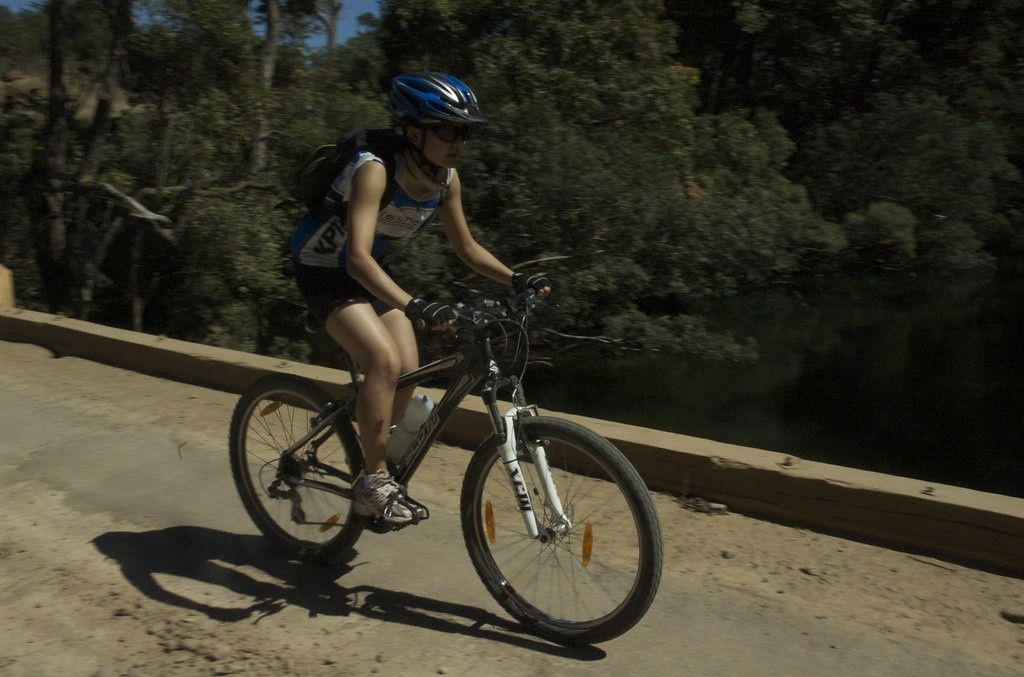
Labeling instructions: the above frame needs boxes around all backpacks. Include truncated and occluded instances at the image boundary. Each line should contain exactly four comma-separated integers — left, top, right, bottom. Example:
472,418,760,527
287,127,447,242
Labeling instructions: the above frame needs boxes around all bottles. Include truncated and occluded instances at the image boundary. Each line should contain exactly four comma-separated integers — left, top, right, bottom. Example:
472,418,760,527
385,394,434,466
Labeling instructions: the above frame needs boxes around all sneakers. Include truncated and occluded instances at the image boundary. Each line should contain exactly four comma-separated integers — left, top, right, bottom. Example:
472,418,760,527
352,469,423,523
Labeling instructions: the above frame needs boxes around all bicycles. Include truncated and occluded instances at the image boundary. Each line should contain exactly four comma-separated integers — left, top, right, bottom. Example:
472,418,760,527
227,287,664,651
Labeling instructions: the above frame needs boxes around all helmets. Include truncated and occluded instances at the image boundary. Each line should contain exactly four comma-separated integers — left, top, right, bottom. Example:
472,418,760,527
388,72,490,125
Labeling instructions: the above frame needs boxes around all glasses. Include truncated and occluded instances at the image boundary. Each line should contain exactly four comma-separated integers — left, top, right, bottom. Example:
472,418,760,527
431,124,472,142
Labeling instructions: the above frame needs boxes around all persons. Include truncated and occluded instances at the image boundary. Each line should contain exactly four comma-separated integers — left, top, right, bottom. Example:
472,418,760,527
292,70,552,529
